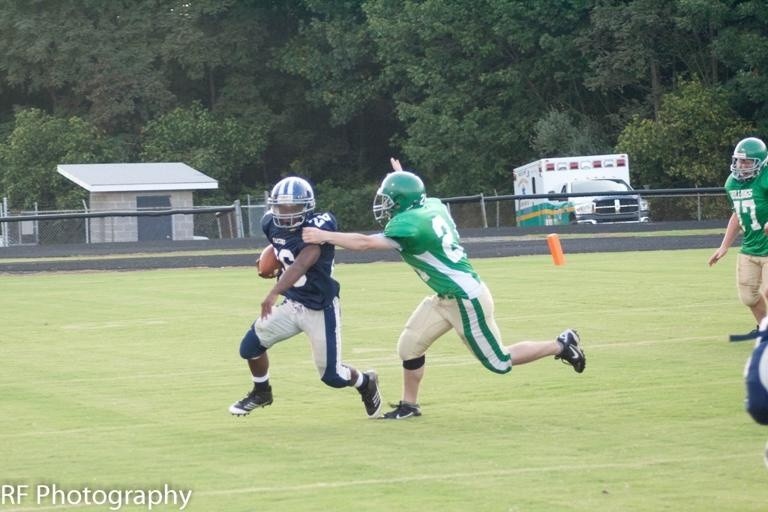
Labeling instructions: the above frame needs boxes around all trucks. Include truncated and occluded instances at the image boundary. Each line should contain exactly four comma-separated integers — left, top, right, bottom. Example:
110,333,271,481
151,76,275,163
513,153,650,225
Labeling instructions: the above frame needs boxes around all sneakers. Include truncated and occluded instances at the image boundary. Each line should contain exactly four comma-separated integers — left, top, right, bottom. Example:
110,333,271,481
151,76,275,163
554,328,586,375
381,400,422,420
357,369,383,419
228,385,273,417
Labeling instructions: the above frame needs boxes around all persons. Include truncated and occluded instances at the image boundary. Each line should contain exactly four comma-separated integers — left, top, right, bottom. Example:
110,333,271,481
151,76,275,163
302,157,585,419
229,176,383,418
730,317,768,467
709,137,768,326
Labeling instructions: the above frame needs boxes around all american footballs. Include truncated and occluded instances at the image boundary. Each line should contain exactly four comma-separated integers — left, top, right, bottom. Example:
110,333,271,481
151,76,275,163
259,244,283,275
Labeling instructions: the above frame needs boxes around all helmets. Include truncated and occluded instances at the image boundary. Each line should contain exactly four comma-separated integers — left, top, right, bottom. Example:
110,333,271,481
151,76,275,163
734,137,768,173
271,176,315,216
379,171,427,219
